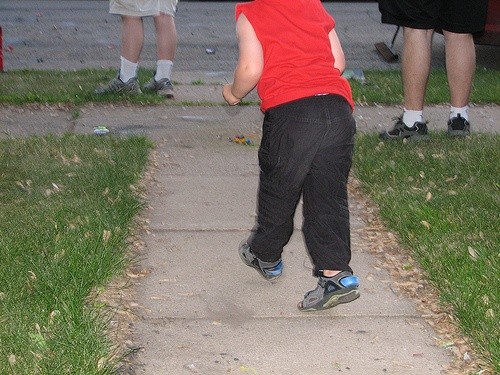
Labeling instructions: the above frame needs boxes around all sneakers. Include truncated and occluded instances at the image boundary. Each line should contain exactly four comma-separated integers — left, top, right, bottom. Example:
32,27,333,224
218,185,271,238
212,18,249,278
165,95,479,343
378,116,430,144
238,237,286,282
95,71,143,97
448,115,471,137
141,75,175,97
296,271,364,313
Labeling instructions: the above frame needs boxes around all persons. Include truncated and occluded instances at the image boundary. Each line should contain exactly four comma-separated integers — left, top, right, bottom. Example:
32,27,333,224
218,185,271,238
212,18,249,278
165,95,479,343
93,0,178,99
377,0,489,142
221,0,361,312
